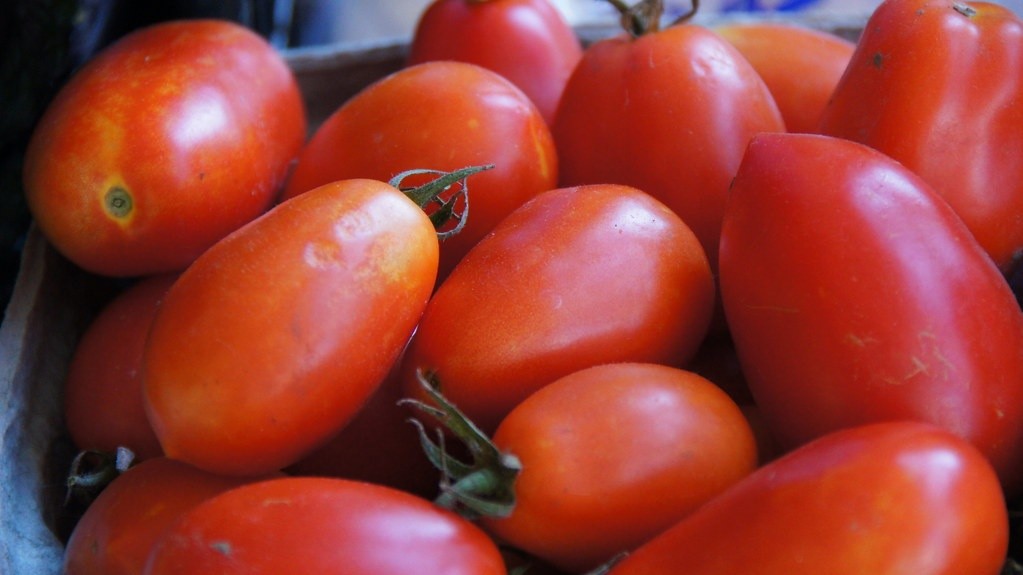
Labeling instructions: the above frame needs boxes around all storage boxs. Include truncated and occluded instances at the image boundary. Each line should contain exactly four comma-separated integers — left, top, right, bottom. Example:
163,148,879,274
0,8,1022,575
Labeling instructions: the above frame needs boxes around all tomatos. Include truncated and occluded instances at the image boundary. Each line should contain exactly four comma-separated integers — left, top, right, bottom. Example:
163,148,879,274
20,0,1023,575
